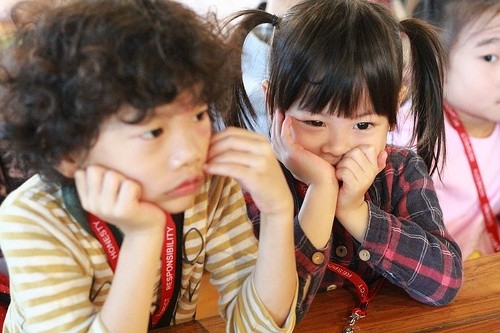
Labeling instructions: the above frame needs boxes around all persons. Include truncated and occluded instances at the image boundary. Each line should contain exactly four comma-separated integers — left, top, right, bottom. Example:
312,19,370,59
213,0,463,325
0,0,500,333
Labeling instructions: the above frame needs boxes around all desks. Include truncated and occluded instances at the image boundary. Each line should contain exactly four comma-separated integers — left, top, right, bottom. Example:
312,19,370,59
148,252,500,333
